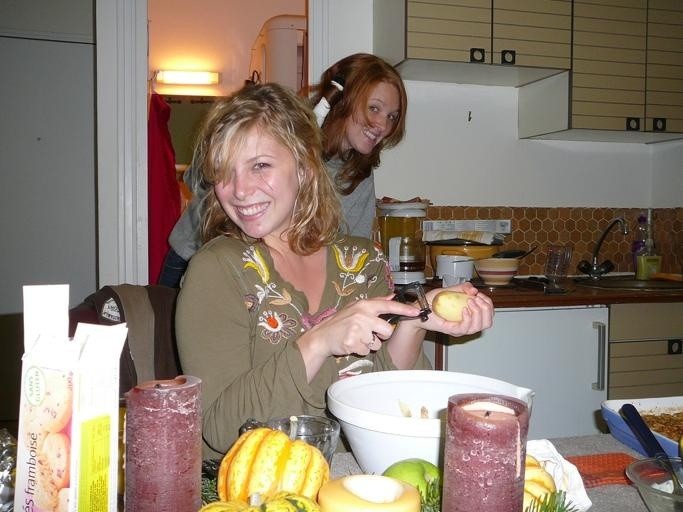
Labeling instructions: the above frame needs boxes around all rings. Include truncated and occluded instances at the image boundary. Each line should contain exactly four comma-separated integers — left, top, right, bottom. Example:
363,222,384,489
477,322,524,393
367,335,376,347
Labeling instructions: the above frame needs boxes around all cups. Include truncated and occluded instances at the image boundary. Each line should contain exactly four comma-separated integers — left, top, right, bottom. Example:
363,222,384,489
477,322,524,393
246,415,341,471
546,246,573,279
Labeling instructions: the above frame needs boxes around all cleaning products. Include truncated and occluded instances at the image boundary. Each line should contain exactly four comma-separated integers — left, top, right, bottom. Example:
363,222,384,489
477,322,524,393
634,225,663,280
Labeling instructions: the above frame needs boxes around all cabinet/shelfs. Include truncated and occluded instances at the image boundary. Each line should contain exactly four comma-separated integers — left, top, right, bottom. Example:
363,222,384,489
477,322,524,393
606,303,683,400
520,2,682,145
373,0,571,87
388,305,610,437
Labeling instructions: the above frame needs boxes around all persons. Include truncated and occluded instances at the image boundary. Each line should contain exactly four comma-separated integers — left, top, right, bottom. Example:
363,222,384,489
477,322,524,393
157,52,406,289
175,82,494,474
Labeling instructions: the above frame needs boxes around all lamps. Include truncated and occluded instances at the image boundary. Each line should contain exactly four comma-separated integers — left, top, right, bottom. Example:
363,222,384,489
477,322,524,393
153,69,221,85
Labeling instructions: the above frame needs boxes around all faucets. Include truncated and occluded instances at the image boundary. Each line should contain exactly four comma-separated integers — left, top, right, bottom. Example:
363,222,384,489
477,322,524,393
579,216,630,280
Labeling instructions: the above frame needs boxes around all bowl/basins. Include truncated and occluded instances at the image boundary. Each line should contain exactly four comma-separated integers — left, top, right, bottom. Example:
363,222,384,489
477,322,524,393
436,254,472,282
624,457,683,512
326,370,532,474
475,258,520,287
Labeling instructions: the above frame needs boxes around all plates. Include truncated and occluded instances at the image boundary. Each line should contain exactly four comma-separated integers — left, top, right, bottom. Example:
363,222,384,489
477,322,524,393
600,395,683,464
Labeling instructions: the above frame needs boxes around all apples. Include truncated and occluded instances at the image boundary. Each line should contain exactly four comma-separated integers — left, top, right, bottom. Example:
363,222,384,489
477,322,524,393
381,458,441,503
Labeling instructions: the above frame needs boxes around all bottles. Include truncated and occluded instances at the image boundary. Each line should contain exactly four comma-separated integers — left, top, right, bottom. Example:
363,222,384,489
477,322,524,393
628,214,649,277
632,238,662,274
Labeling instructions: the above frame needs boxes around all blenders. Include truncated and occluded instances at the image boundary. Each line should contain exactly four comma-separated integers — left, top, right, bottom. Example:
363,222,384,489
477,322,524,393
377,201,426,286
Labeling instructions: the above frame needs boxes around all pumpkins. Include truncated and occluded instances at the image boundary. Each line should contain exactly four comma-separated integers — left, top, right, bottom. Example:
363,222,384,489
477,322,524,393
522,455,556,512
217,428,329,505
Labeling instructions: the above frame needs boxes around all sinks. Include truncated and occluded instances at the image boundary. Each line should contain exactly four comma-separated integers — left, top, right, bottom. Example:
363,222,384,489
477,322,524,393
581,278,683,290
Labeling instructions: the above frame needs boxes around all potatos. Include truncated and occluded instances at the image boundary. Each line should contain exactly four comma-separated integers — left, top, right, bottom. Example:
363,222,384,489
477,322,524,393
430,290,478,324
